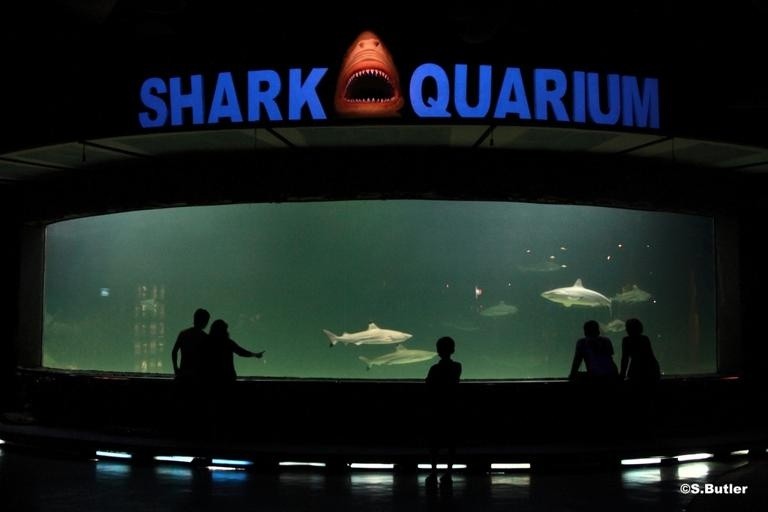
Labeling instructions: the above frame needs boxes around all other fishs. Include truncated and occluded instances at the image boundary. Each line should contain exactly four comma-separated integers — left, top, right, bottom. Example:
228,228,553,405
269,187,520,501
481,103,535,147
517,240,655,277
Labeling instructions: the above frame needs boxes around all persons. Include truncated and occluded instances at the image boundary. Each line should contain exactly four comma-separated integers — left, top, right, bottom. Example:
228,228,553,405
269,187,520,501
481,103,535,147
171,307,208,391
424,335,462,485
567,318,620,384
620,318,662,386
197,318,267,386
